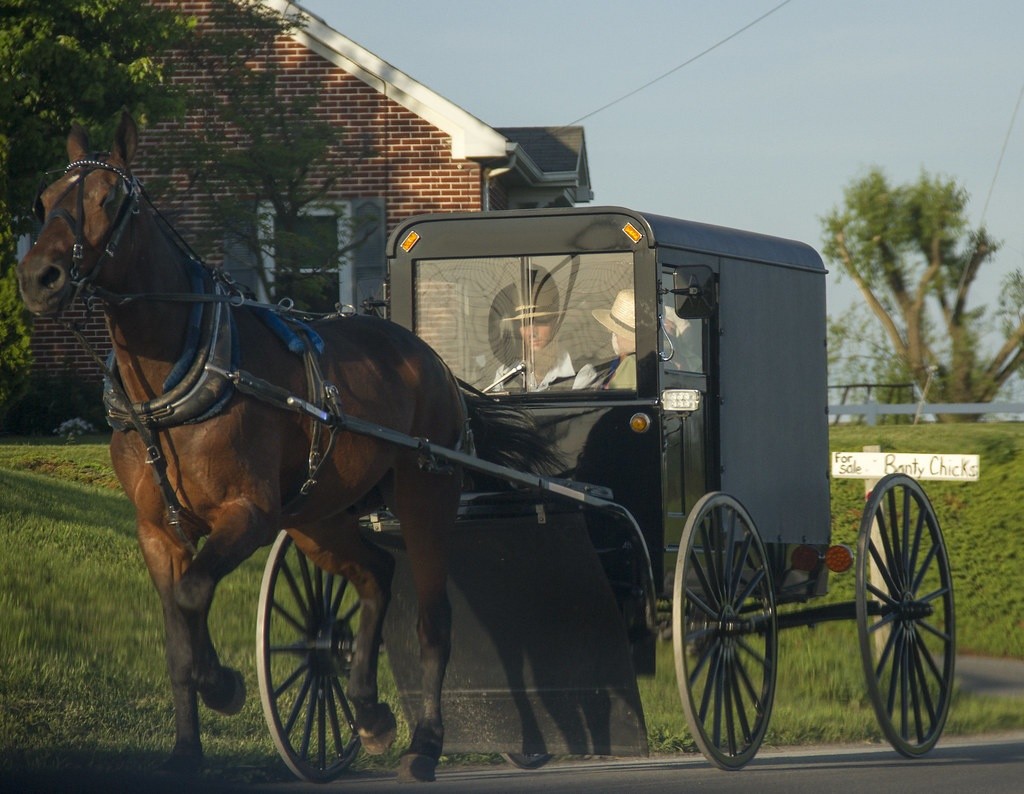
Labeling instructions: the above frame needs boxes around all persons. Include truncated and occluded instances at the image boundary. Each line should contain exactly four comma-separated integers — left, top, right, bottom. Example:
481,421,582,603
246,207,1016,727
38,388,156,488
662,306,703,373
572,288,637,391
473,288,587,392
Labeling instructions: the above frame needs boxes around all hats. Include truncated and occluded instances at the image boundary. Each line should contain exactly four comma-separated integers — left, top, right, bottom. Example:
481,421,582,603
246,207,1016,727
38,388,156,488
591,289,636,339
494,285,566,321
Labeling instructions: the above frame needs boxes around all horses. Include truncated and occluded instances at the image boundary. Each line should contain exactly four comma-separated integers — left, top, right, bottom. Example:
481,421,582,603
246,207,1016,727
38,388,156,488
14,107,572,794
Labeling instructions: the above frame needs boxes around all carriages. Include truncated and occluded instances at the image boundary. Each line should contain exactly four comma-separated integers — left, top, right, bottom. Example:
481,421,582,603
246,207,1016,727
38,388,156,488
22,108,956,782
